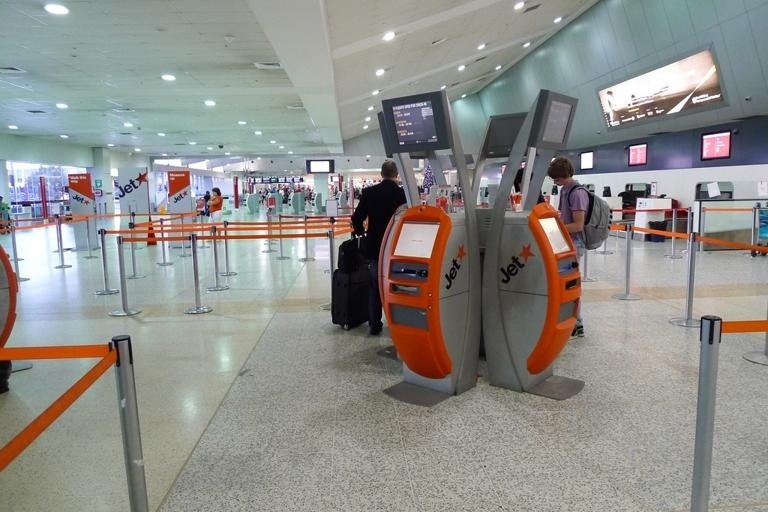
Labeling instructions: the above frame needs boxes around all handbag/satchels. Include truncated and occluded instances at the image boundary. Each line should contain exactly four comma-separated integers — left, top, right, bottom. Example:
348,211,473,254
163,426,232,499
337,230,370,273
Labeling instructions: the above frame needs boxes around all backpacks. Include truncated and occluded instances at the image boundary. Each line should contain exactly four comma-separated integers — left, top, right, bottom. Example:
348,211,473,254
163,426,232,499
565,184,614,250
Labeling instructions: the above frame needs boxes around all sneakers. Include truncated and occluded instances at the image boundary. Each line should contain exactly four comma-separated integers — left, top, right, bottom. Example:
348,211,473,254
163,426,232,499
569,325,586,339
370,321,384,335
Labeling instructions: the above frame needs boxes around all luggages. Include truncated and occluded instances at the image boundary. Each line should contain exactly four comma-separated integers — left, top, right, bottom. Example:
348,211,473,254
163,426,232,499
331,267,370,331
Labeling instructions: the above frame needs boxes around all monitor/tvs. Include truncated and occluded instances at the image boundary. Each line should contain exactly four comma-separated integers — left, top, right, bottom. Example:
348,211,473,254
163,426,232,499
484,112,529,159
501,157,558,175
528,88,578,150
579,151,593,170
628,144,646,166
306,158,336,175
377,111,429,160
700,130,732,161
380,90,453,156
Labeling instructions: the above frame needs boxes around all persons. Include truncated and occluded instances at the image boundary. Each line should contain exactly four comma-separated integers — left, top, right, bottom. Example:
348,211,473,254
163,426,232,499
351,161,408,336
514,168,545,206
547,157,590,340
0,196,12,234
207,187,224,242
256,179,373,205
416,182,490,199
203,191,211,216
